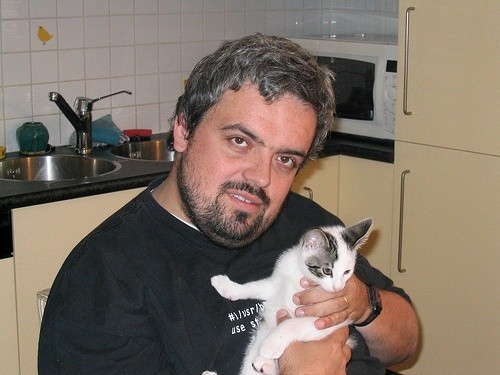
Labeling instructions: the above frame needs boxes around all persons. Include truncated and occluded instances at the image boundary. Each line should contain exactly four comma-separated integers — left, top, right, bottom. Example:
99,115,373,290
37,34,421,375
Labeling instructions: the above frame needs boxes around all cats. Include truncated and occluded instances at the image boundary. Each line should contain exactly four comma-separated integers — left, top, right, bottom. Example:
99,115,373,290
198,218,373,375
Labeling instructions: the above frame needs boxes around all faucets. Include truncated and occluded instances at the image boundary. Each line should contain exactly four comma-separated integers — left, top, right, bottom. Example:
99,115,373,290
47,90,95,156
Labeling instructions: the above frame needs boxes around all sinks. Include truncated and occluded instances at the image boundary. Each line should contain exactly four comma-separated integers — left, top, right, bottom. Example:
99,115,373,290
0,153,122,185
107,137,182,163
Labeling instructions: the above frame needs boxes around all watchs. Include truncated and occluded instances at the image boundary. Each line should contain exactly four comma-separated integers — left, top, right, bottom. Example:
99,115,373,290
355,284,382,327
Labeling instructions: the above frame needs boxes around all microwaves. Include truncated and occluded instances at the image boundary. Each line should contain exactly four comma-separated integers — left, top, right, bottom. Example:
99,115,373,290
282,32,399,141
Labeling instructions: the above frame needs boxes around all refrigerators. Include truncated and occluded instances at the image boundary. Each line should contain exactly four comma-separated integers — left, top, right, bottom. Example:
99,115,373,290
375,0,500,375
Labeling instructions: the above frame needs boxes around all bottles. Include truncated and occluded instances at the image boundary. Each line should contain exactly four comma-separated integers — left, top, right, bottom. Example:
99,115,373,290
16,122,49,152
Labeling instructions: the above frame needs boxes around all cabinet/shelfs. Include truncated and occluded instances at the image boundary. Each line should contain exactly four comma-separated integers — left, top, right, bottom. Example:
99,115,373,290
0,0,500,375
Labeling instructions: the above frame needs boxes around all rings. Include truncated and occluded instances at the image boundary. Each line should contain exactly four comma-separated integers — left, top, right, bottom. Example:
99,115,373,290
344,297,347,308
